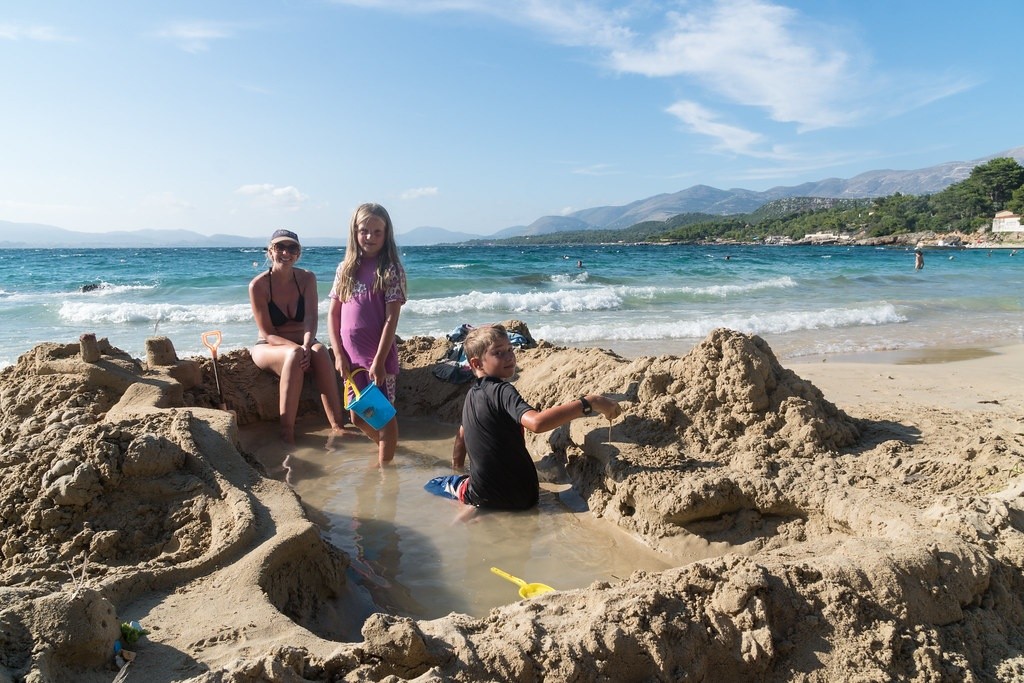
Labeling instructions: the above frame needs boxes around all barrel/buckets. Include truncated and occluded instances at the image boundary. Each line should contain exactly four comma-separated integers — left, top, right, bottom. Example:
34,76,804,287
344,368,398,431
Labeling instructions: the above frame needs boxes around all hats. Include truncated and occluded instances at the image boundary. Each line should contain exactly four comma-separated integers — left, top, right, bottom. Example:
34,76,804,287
271,229,298,246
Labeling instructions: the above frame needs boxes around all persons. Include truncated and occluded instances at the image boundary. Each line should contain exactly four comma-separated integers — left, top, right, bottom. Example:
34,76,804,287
915,250,924,269
574,261,584,267
328,203,408,470
247,229,343,432
421,323,622,525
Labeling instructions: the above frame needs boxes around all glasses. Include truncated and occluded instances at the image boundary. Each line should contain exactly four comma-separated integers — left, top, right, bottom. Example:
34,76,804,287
273,244,299,255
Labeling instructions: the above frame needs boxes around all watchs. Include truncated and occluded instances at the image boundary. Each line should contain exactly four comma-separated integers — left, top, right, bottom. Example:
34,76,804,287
579,397,592,415
